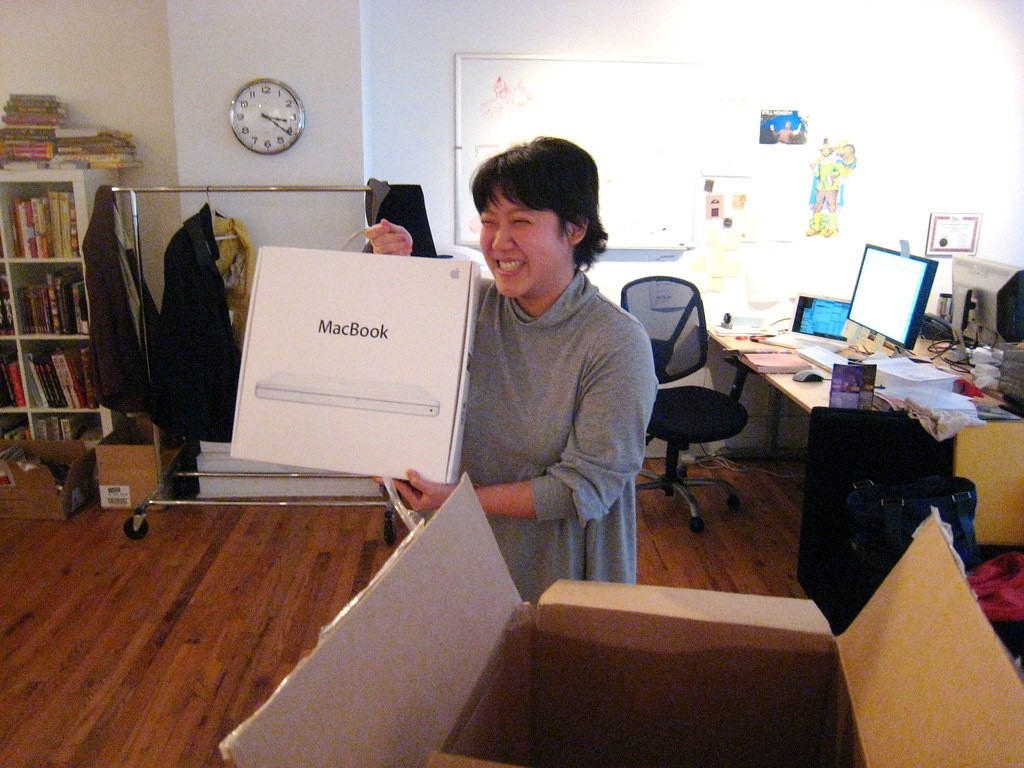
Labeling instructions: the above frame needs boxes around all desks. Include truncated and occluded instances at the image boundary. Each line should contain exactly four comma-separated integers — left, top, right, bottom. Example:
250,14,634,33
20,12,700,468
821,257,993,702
706,326,1024,464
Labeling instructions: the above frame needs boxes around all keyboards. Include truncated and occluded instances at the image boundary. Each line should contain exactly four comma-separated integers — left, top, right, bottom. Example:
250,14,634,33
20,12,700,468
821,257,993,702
796,346,850,373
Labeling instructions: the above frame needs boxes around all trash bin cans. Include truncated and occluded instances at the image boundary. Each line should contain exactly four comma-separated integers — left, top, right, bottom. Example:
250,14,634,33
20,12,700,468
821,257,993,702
798,409,951,636
845,475,981,614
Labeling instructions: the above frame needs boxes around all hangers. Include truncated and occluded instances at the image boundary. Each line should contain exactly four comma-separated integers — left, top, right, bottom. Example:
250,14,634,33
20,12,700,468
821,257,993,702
207,185,238,242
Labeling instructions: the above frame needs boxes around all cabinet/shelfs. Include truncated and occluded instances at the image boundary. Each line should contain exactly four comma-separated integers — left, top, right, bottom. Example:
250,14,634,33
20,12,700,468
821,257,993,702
1,169,121,451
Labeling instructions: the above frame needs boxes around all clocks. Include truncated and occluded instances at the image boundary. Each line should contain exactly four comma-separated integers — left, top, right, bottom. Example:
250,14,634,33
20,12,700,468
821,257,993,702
228,76,305,155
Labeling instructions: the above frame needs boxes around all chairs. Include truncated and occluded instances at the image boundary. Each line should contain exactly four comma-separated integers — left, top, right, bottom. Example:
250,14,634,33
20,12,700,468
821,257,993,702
794,408,1024,658
619,275,748,535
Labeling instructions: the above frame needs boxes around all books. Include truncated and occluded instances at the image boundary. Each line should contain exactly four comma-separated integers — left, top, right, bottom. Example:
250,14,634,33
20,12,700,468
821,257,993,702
1,94,143,486
723,347,811,373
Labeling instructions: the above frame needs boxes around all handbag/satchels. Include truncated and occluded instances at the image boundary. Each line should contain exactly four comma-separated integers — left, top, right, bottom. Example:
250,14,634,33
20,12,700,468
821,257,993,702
232,226,481,484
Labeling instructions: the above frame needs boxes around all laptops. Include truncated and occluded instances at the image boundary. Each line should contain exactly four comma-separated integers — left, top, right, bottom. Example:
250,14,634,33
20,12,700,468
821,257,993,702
762,293,852,353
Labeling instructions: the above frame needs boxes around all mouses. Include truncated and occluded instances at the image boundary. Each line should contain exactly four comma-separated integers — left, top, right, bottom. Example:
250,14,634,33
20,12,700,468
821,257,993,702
793,369,824,382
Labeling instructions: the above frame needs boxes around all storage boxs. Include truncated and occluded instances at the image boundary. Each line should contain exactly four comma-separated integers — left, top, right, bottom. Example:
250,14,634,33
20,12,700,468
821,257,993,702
95,416,178,512
230,244,481,485
0,438,96,521
216,472,1023,768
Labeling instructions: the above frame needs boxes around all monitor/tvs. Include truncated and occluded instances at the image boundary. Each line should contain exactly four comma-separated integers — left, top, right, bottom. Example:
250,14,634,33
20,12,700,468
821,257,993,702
847,244,939,351
944,255,1024,365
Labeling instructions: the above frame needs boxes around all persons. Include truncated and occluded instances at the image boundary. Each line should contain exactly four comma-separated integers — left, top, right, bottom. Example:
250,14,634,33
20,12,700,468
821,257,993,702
364,137,659,606
